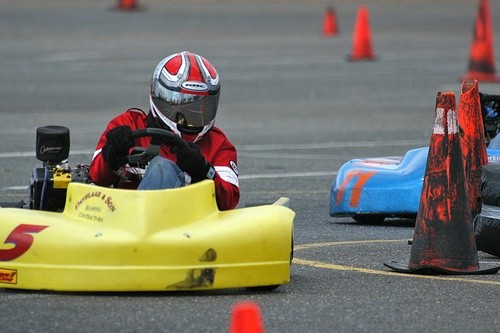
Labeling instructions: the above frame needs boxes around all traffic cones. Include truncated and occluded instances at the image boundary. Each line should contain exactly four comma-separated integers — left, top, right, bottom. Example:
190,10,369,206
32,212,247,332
460,0,499,82
345,6,377,61
230,302,265,332
322,6,339,37
112,0,139,10
385,78,499,275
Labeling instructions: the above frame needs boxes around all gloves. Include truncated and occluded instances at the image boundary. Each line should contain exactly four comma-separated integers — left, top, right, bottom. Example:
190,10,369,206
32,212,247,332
171,142,215,184
102,125,135,171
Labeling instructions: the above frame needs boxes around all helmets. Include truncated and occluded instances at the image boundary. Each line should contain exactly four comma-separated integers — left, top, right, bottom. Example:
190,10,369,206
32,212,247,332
149,51,220,143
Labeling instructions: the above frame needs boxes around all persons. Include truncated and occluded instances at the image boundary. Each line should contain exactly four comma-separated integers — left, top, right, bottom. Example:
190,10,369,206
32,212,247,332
89,50,240,211
